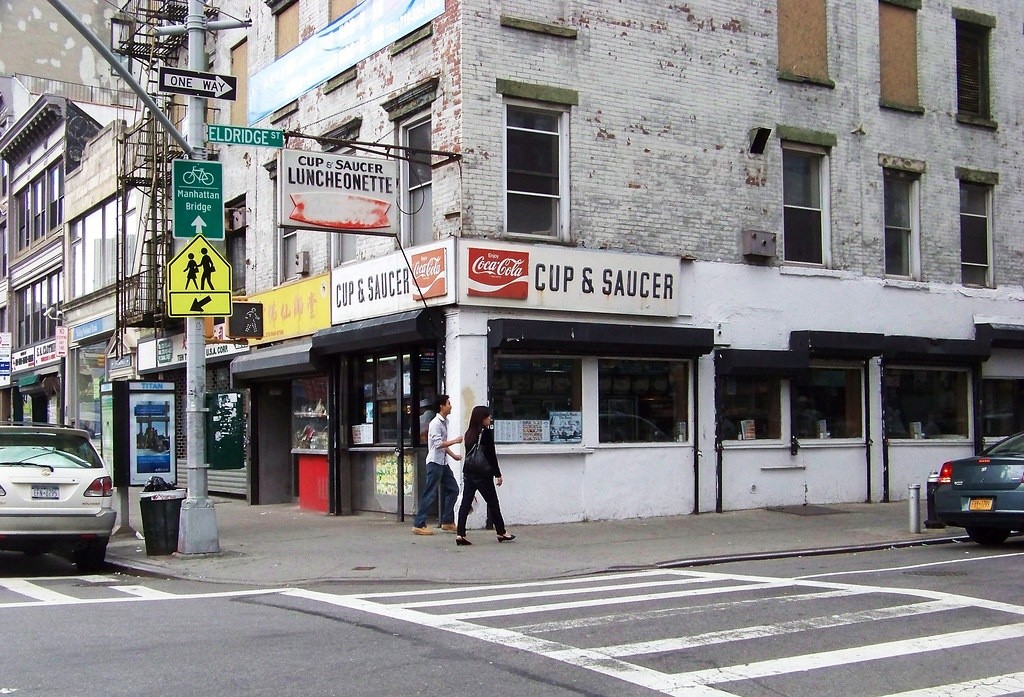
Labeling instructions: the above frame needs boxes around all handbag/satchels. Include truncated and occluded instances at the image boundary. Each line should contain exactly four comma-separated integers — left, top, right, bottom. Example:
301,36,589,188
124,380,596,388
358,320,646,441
463,429,492,476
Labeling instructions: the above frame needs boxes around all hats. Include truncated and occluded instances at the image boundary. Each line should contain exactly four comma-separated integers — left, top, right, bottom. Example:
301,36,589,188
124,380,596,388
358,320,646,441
420,398,432,407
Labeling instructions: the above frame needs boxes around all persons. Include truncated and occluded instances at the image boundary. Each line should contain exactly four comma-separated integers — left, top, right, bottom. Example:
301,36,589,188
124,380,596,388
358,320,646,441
456,405,517,546
411,395,464,534
408,399,437,446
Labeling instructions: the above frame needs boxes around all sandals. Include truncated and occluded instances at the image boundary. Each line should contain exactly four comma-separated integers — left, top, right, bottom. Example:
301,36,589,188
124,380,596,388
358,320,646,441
496,532,516,543
455,535,471,546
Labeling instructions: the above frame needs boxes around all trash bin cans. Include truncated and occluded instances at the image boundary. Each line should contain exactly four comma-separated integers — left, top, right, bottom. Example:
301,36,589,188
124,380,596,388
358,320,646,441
138,489,187,557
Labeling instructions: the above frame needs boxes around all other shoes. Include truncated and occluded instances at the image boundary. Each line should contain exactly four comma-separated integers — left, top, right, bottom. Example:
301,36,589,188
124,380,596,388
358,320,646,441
441,522,458,531
412,526,434,535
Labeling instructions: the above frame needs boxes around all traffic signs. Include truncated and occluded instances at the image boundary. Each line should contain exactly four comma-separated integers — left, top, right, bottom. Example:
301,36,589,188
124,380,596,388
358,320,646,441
157,65,239,102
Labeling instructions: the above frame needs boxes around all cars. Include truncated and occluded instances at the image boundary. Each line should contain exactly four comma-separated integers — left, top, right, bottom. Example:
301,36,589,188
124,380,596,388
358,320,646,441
0,421,118,575
932,431,1024,546
